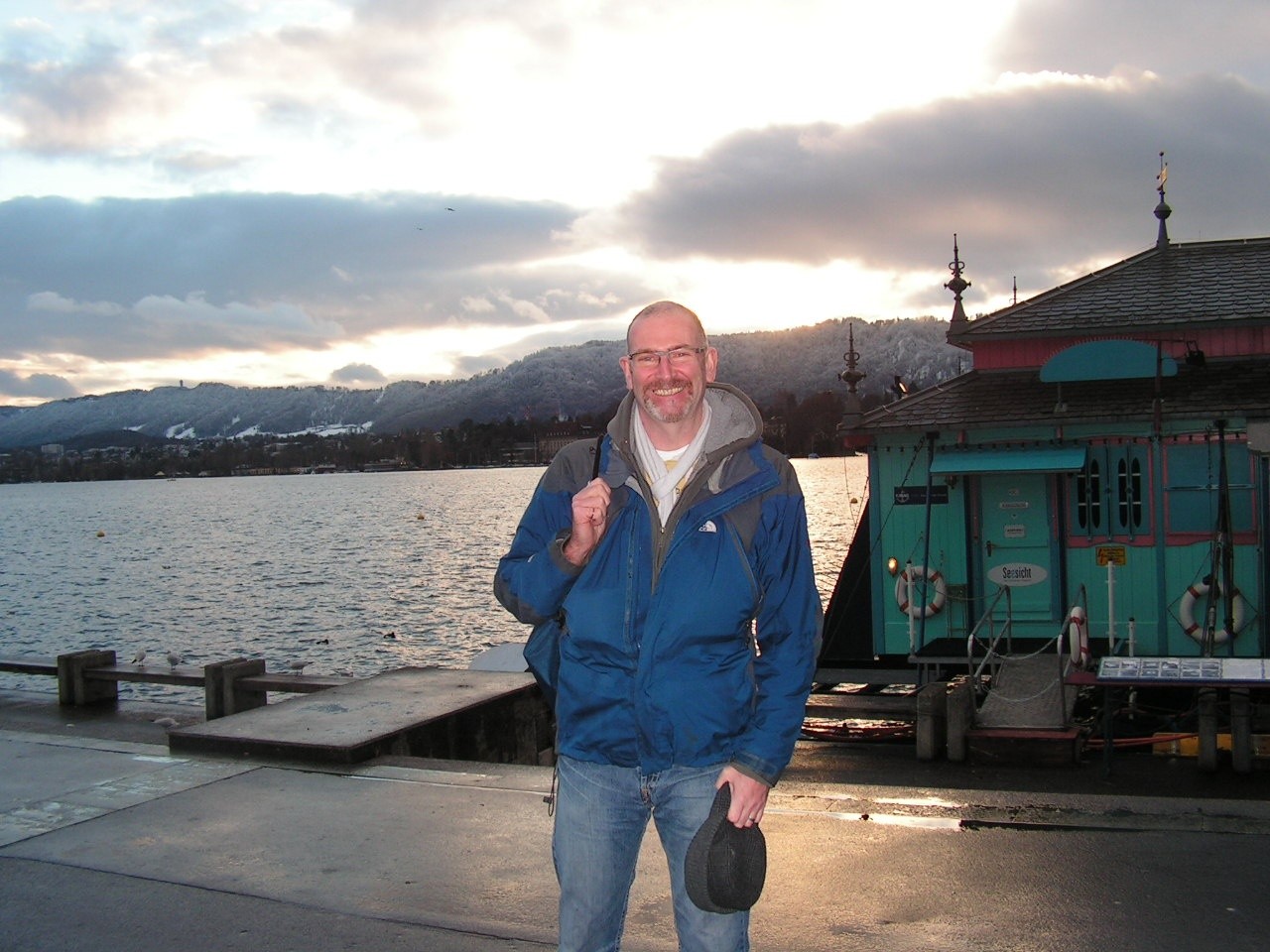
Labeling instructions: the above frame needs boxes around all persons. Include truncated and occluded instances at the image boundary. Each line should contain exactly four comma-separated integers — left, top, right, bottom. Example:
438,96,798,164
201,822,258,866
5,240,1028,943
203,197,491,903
493,300,824,951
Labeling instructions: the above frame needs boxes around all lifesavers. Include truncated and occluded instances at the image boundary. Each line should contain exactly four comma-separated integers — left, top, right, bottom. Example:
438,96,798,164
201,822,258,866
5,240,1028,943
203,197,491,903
895,566,946,618
1069,607,1088,668
1180,582,1244,643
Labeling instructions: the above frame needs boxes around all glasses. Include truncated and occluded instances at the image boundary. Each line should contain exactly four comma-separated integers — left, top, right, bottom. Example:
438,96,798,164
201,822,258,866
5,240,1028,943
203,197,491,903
628,346,708,370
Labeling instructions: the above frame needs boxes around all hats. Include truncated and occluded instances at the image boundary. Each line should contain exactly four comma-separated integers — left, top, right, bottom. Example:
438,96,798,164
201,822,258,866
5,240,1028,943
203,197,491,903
684,781,768,914
